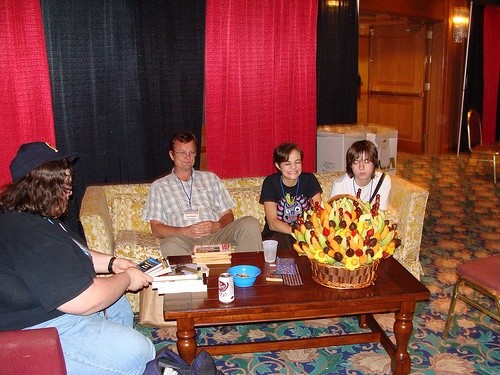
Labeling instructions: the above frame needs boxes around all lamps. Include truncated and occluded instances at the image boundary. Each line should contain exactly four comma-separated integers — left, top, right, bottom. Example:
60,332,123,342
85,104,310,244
453,7,470,43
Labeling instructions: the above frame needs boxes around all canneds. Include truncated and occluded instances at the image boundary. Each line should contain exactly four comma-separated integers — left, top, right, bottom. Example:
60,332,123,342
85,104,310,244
217,273,235,304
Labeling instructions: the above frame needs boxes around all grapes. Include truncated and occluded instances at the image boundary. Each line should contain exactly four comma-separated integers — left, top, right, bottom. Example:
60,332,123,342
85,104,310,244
295,220,401,261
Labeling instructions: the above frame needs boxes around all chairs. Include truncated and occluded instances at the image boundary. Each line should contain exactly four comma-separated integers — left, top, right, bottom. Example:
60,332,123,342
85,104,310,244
465,108,500,184
0,327,67,375
442,254,500,339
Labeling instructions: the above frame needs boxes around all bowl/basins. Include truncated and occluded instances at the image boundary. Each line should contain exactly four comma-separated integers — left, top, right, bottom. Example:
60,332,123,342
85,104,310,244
226,265,261,287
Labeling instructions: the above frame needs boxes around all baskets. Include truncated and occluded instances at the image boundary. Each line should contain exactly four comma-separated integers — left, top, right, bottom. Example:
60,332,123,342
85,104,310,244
309,194,381,290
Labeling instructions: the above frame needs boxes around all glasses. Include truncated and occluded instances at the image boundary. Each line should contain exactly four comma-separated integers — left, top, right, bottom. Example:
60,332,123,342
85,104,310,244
172,151,197,159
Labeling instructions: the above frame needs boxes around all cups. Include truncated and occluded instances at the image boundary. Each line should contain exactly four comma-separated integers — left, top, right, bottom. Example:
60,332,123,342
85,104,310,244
262,240,278,263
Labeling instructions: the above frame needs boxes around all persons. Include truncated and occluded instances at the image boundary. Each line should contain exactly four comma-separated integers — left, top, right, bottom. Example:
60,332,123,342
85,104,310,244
141,130,263,260
259,143,323,238
328,140,392,211
0,142,156,375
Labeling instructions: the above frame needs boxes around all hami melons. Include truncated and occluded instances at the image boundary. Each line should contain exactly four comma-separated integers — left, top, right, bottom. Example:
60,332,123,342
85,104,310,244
294,201,396,271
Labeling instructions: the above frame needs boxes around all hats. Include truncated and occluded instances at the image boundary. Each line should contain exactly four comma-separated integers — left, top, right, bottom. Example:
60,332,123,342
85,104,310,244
9,142,80,183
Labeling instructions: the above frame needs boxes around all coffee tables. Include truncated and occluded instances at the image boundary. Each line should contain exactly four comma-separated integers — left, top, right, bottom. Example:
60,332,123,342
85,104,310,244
164,249,431,375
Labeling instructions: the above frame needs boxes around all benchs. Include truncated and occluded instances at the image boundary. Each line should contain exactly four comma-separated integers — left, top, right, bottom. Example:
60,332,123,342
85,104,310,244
78,170,429,317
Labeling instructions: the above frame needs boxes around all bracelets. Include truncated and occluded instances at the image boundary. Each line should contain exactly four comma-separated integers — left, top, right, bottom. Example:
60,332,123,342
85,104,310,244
108,257,116,272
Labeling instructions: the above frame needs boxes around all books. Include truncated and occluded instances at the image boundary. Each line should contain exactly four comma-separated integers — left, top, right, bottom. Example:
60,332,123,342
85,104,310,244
151,264,209,294
191,243,232,264
135,256,172,277
